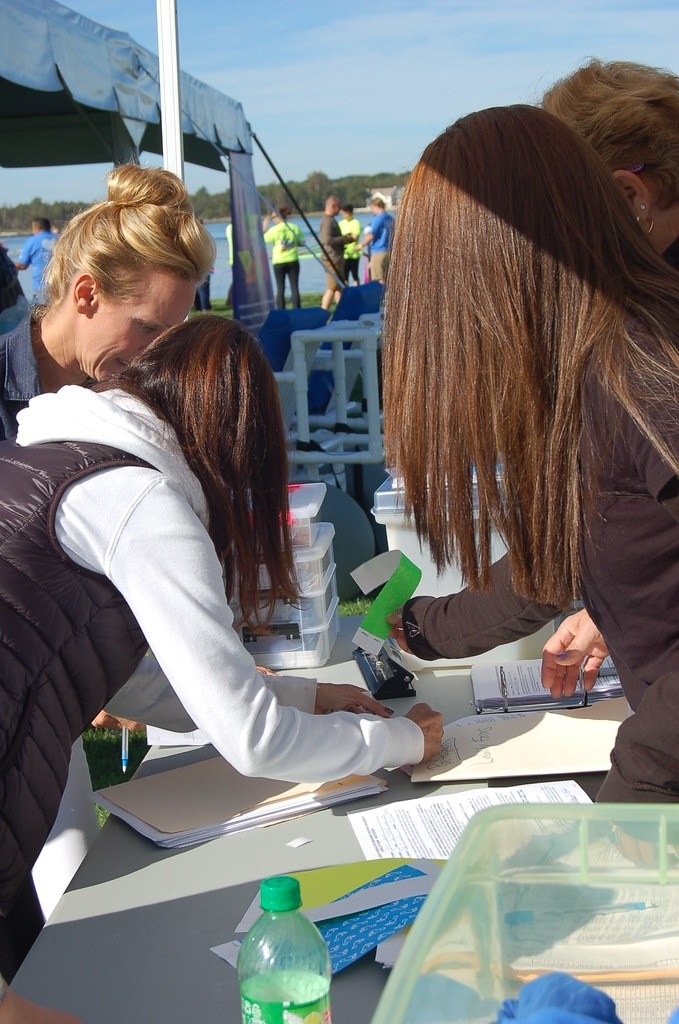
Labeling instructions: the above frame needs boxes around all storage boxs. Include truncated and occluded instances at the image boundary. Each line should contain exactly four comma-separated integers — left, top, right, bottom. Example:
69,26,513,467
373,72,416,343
369,471,556,669
364,802,678,1024
234,486,342,671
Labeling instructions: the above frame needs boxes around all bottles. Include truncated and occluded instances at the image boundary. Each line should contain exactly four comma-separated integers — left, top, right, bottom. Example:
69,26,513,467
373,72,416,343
235,876,332,1024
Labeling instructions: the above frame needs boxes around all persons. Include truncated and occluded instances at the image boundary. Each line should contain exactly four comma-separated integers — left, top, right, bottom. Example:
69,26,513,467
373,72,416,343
1,316,443,984
383,104,678,868
0,163,215,438
225,198,394,308
0,217,61,333
541,59,679,698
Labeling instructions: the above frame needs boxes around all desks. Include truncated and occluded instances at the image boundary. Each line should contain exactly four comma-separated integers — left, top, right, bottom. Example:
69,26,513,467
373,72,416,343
0,618,679,1024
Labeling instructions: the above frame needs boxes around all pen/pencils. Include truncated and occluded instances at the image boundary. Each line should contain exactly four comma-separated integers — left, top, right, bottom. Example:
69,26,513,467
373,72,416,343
392,626,418,634
499,666,509,711
503,900,657,924
120,726,129,774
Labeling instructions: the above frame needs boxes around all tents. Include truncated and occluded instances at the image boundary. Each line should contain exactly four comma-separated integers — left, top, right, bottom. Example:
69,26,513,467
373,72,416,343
0,0,348,288
